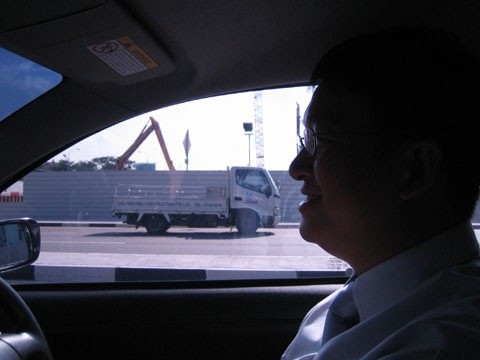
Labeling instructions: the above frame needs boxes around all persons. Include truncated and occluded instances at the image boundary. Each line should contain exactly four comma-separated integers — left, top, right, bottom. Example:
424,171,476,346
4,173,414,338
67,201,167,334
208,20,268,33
280,25,480,360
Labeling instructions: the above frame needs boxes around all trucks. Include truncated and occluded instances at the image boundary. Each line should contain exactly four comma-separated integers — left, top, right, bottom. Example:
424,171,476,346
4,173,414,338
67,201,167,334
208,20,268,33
113,167,283,236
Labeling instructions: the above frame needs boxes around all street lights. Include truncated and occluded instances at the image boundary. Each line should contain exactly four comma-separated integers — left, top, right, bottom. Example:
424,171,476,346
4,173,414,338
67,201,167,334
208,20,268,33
242,121,254,167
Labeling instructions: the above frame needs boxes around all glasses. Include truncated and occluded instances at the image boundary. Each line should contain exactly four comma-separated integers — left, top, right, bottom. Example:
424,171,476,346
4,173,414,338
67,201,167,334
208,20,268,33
296,128,381,156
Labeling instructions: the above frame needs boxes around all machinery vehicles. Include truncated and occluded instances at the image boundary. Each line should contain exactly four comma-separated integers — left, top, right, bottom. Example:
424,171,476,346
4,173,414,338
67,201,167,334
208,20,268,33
115,117,176,173
